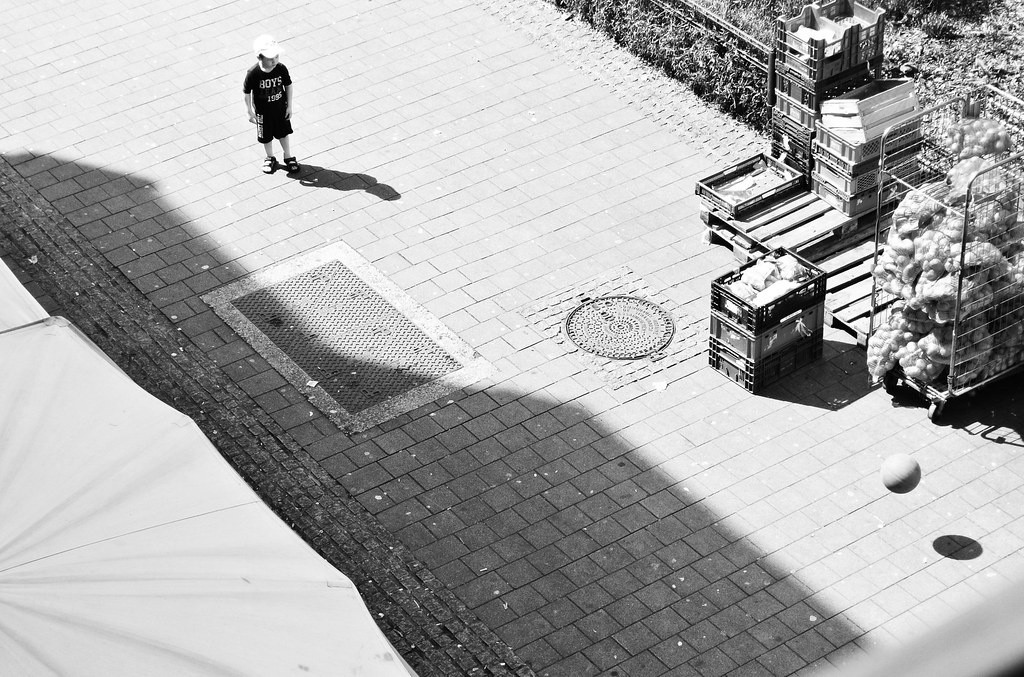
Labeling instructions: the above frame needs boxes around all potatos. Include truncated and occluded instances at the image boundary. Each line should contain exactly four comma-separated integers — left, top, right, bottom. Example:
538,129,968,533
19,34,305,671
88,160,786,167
867,121,1023,386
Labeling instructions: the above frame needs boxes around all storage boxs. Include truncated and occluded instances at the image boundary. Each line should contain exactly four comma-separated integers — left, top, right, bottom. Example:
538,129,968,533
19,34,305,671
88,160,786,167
711,245,827,337
710,302,824,363
708,328,824,394
694,0,922,220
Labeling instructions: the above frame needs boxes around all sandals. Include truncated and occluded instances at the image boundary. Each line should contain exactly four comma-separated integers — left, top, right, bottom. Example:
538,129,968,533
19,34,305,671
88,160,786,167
283,156,300,173
263,156,276,173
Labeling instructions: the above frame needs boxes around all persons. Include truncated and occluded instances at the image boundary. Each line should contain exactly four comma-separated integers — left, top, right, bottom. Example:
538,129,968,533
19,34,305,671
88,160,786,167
242,35,301,174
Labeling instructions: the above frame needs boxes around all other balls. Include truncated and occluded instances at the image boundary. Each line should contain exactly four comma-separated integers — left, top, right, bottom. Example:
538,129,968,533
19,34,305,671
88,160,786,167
880,453,922,495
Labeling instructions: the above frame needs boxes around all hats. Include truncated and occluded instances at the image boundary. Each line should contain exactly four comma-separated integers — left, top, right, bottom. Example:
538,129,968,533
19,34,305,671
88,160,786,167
254,36,281,58
